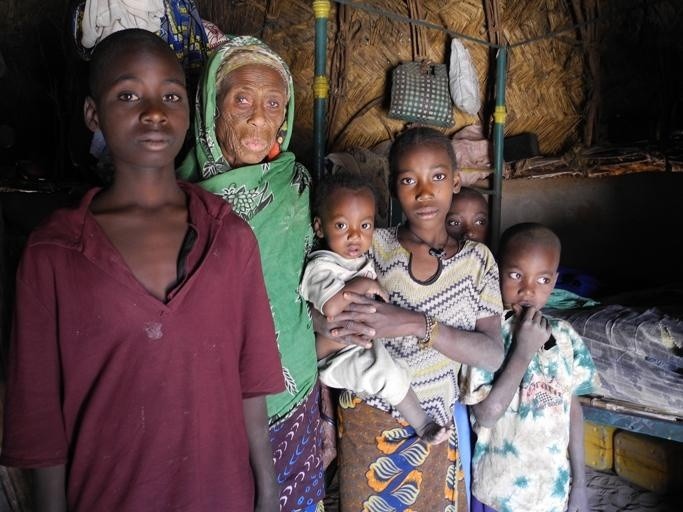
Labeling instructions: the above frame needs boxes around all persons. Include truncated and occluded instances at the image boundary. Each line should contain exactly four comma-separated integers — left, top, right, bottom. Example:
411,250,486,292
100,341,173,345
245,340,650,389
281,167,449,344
295,172,456,447
310,125,507,511
0,26,290,510
445,186,491,243
469,222,603,511
173,33,340,510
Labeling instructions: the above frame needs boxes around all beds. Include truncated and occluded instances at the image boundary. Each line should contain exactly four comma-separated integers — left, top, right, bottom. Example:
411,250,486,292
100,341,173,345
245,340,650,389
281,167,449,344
311,0,682,448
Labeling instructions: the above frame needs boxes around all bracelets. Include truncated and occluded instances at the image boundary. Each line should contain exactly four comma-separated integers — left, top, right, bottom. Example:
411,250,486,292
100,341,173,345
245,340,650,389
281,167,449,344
320,414,337,427
416,311,440,354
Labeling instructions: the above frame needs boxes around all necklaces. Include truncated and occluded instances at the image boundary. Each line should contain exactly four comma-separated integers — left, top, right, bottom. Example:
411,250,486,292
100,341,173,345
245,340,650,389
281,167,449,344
405,228,450,258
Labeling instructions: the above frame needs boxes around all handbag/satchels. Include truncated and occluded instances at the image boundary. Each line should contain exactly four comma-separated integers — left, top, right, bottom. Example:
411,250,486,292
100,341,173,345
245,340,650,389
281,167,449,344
446,40,483,114
389,60,457,130
